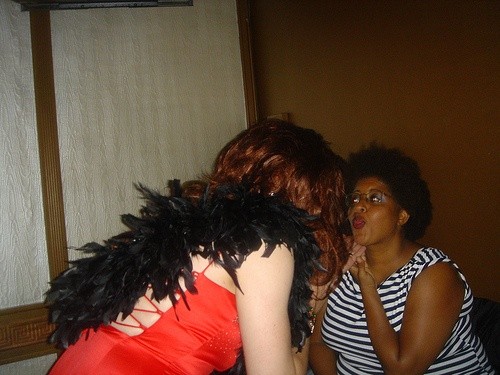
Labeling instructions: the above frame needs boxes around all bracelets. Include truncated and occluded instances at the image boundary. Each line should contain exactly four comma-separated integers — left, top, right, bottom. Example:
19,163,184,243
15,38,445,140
307,307,316,332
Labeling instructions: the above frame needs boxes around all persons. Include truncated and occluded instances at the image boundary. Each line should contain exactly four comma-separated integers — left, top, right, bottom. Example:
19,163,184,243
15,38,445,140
308,145,497,375
47,118,354,375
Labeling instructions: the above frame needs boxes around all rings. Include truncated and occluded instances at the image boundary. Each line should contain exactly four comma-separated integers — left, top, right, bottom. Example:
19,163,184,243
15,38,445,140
348,252,355,256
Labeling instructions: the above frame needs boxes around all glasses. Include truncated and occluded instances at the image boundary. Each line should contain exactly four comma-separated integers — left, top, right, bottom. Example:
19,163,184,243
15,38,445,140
344,190,393,208
